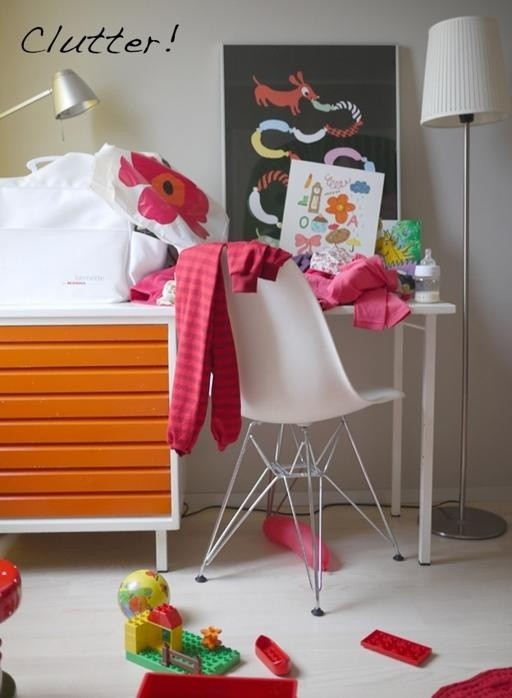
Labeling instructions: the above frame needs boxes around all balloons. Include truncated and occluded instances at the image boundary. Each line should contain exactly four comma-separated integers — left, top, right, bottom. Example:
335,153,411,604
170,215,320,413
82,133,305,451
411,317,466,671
262,515,330,571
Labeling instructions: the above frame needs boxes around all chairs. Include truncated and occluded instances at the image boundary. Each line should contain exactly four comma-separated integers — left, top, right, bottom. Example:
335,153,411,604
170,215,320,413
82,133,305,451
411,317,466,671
174,240,407,620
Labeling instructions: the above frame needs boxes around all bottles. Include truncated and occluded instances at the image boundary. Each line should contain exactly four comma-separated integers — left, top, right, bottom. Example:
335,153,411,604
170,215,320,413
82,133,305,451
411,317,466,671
413,248,441,305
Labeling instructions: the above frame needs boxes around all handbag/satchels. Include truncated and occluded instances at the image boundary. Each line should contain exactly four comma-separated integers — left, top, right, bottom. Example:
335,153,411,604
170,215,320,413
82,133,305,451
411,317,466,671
0,144,168,310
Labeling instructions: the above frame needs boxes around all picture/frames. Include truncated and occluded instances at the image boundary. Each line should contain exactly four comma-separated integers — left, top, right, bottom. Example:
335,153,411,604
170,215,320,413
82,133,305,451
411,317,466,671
218,41,400,244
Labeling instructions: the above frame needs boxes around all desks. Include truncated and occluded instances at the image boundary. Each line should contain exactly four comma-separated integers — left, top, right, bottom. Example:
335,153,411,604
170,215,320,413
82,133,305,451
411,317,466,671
1,299,456,568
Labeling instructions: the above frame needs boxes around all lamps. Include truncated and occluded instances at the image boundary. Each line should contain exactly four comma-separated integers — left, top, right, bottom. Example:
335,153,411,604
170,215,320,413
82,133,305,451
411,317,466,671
420,12,511,543
1,67,99,124
